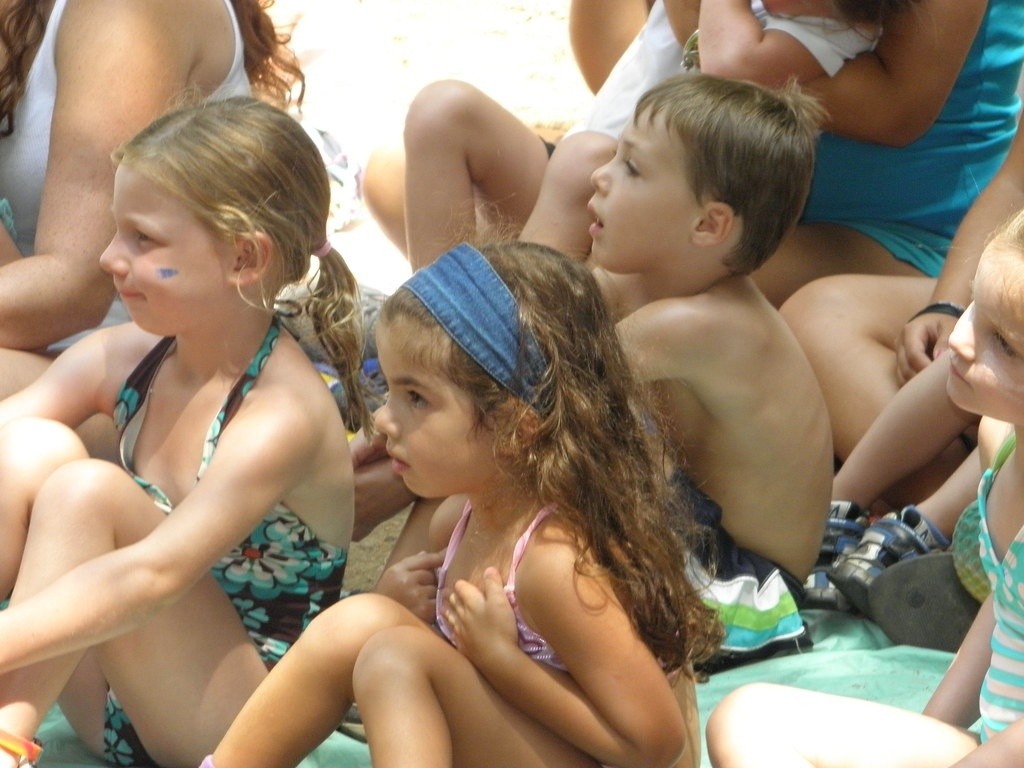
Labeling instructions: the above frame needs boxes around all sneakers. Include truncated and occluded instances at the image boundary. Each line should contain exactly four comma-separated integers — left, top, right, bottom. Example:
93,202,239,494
802,499,870,610
826,505,950,612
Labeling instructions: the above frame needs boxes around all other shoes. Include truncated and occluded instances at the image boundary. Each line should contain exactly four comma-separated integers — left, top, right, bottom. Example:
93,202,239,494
312,359,392,443
867,551,983,652
286,281,393,360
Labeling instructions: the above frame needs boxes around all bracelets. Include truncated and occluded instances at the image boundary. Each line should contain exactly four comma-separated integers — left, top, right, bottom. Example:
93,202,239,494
910,302,968,317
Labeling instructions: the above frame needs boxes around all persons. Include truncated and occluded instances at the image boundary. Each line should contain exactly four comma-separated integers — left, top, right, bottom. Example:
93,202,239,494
195,239,728,768
0,0,1024,768
